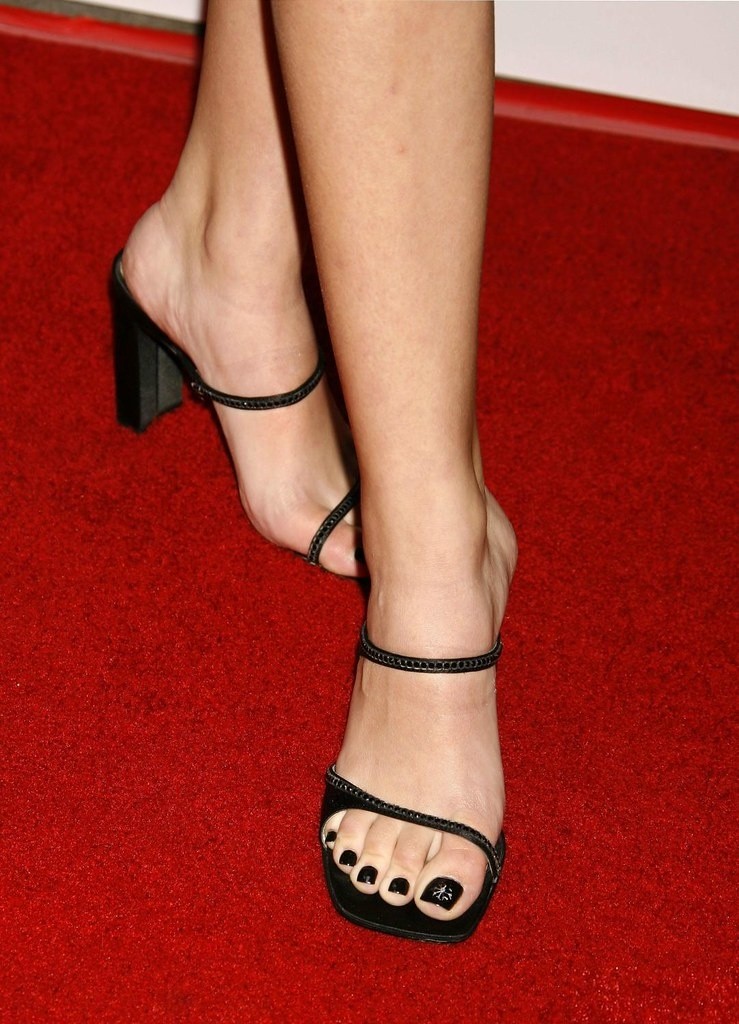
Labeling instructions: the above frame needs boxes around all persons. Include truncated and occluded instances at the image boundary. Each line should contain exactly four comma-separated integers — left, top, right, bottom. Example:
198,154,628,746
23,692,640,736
105,0,519,949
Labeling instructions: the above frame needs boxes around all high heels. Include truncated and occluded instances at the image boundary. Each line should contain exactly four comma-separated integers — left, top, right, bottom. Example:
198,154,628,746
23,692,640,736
320,606,507,942
106,250,368,582
